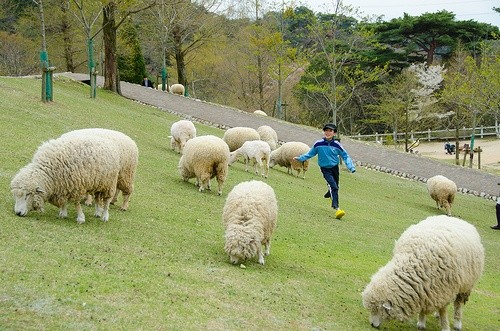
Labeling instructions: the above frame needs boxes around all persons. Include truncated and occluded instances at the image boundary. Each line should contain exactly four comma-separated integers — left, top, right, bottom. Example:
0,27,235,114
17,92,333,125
490,195,500,229
142,76,154,89
293,123,356,218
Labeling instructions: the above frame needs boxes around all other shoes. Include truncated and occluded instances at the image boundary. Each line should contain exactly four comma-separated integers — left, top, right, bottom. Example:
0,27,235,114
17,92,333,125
328,196,333,204
335,209,345,218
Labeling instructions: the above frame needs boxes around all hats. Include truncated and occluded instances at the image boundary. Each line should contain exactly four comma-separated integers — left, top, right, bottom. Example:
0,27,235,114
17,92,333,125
323,124,338,134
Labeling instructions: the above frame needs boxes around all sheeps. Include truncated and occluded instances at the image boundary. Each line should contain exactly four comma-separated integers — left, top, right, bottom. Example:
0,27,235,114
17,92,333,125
10,129,139,224
167,119,310,196
222,180,278,265
362,214,485,331
426,175,458,217
254,110,267,116
154,84,184,97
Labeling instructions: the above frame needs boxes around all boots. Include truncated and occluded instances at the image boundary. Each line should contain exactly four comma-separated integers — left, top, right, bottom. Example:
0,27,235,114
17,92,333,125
489,203,500,230
